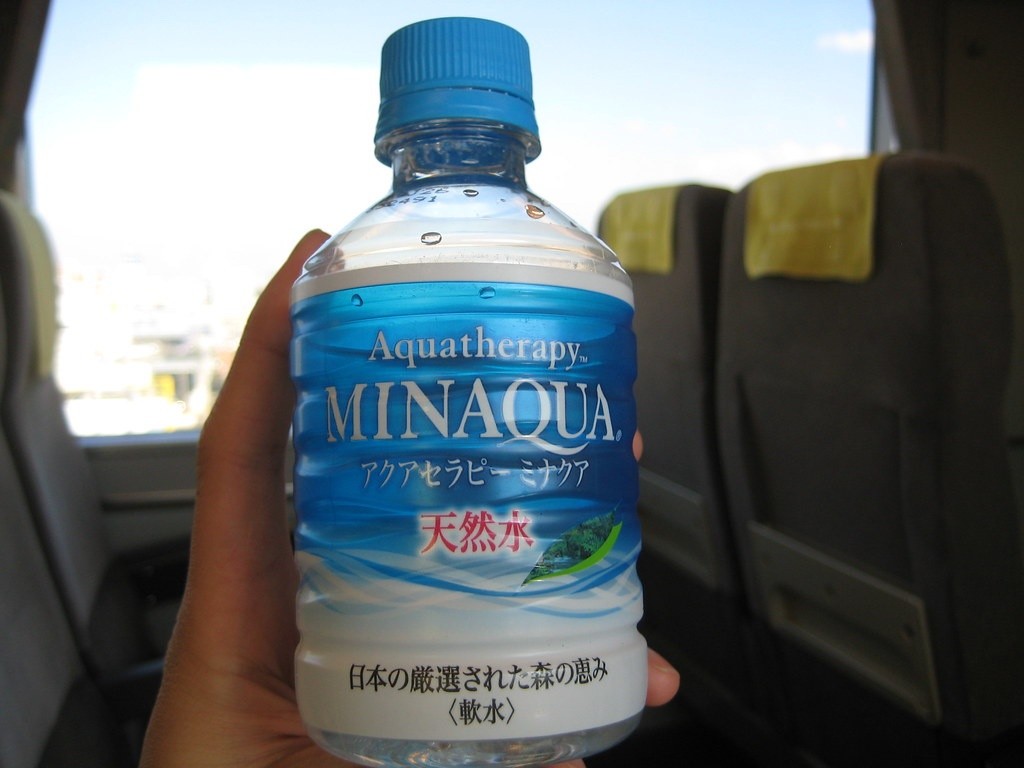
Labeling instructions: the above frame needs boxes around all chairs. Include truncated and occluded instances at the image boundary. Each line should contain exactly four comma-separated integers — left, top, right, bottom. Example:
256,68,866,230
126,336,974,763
596,151,1024,744
0,185,184,768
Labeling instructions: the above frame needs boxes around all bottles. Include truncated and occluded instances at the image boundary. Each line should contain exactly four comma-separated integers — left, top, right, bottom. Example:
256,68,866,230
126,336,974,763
292,16,646,768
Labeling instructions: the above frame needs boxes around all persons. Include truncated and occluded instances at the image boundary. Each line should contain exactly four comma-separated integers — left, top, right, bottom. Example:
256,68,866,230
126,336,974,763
134,229,680,768
